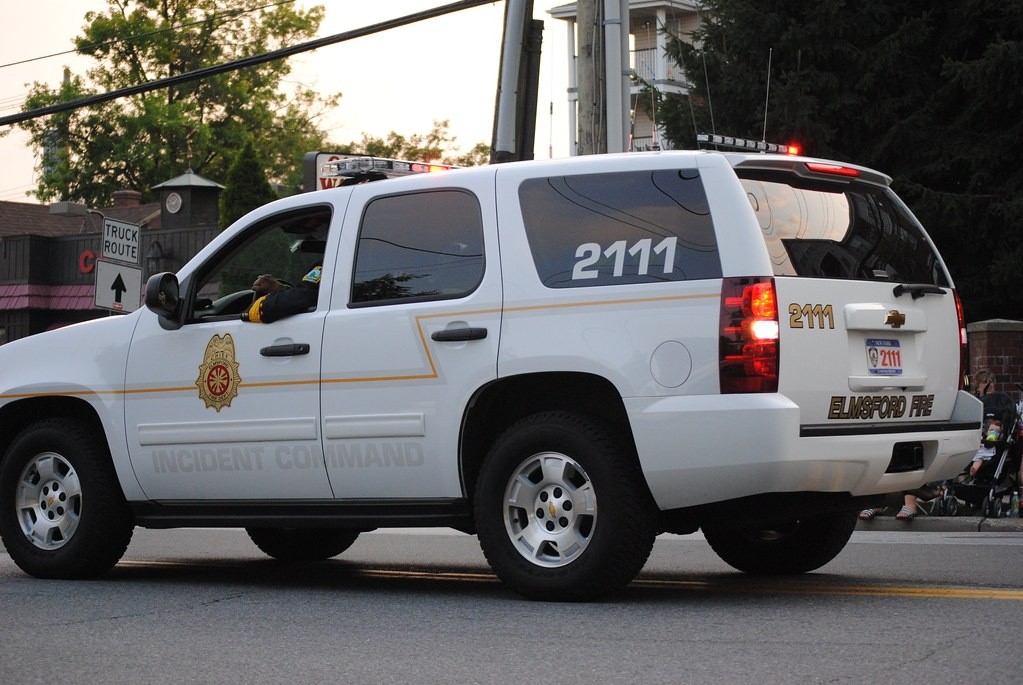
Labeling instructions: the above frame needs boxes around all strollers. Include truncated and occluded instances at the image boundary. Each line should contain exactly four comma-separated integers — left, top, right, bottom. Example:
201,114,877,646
936,370,1023,519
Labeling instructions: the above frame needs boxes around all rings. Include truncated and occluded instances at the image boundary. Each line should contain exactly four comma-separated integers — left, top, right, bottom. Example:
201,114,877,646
258,275,261,278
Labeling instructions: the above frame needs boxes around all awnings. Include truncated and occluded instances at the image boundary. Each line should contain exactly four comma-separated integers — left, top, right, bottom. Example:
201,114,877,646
0,281,225,310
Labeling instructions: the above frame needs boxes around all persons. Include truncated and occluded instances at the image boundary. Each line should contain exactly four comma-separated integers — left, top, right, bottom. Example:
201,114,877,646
241,234,325,324
859,370,1023,521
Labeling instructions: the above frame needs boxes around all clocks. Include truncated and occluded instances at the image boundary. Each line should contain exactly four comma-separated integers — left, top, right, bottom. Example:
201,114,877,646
166,193,182,215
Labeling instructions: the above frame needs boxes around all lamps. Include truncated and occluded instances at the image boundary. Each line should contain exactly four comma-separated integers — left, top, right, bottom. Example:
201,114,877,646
147,241,167,275
50,202,105,233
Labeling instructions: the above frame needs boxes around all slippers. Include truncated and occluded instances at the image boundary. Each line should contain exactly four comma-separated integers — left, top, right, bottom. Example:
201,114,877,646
859,508,882,520
896,505,919,520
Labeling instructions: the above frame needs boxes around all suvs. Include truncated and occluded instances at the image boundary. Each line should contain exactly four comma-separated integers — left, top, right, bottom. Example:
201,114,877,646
0,148,985,582
